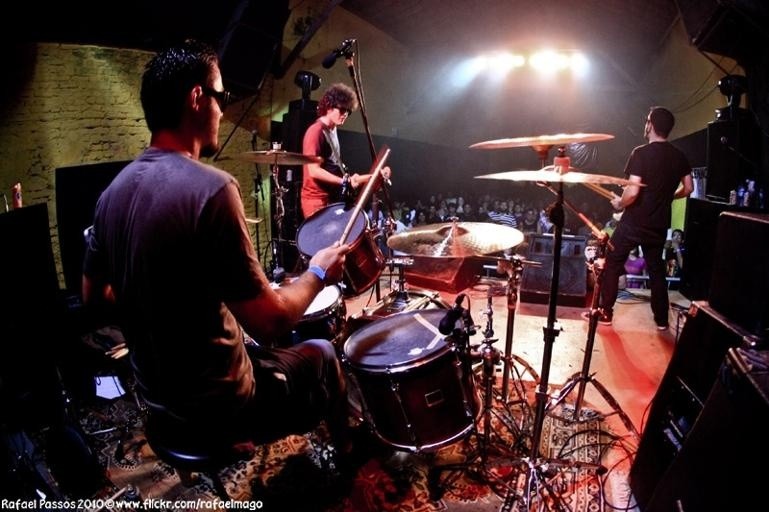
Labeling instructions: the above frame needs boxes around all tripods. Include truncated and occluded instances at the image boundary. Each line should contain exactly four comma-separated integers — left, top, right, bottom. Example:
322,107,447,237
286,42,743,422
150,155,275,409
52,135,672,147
429,186,642,512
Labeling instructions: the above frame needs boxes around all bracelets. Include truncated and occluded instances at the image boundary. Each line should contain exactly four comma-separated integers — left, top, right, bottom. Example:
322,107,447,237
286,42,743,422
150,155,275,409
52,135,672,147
307,265,326,291
341,173,351,195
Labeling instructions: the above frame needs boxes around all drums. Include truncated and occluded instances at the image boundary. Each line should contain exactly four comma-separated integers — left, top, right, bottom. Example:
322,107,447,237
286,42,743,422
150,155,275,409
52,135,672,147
269,276,346,345
346,287,453,337
295,202,386,299
341,308,482,452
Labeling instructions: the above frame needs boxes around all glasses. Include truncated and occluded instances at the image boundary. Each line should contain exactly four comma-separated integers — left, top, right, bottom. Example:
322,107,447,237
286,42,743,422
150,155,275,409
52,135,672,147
202,83,232,114
333,103,354,118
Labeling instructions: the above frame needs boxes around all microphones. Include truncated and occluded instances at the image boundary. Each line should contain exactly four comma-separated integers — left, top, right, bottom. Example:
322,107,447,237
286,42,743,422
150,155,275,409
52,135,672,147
322,39,356,69
439,294,464,335
271,239,285,283
342,164,350,200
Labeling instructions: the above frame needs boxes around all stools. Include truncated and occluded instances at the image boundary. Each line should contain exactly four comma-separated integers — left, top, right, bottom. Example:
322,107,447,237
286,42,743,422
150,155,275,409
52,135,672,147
132,417,268,510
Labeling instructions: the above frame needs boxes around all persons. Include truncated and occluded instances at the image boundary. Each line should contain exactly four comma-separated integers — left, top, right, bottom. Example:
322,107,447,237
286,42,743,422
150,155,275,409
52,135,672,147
82,38,369,458
581,107,695,331
300,84,391,220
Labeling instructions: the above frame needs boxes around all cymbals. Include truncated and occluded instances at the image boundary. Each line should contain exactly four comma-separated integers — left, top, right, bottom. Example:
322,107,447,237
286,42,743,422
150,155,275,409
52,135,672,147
387,215,525,258
473,165,648,187
217,149,324,165
469,133,615,149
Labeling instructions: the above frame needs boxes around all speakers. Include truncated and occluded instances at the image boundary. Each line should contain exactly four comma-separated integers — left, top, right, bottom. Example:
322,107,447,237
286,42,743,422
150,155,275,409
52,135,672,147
629,301,769,512
404,255,482,293
520,233,589,304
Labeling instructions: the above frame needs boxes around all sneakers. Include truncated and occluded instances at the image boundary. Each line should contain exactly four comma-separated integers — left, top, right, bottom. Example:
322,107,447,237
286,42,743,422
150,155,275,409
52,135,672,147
579,306,614,326
655,323,670,332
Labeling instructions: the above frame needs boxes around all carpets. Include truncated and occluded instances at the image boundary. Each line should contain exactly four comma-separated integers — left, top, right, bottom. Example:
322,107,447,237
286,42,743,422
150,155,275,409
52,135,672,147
72,372,613,511
